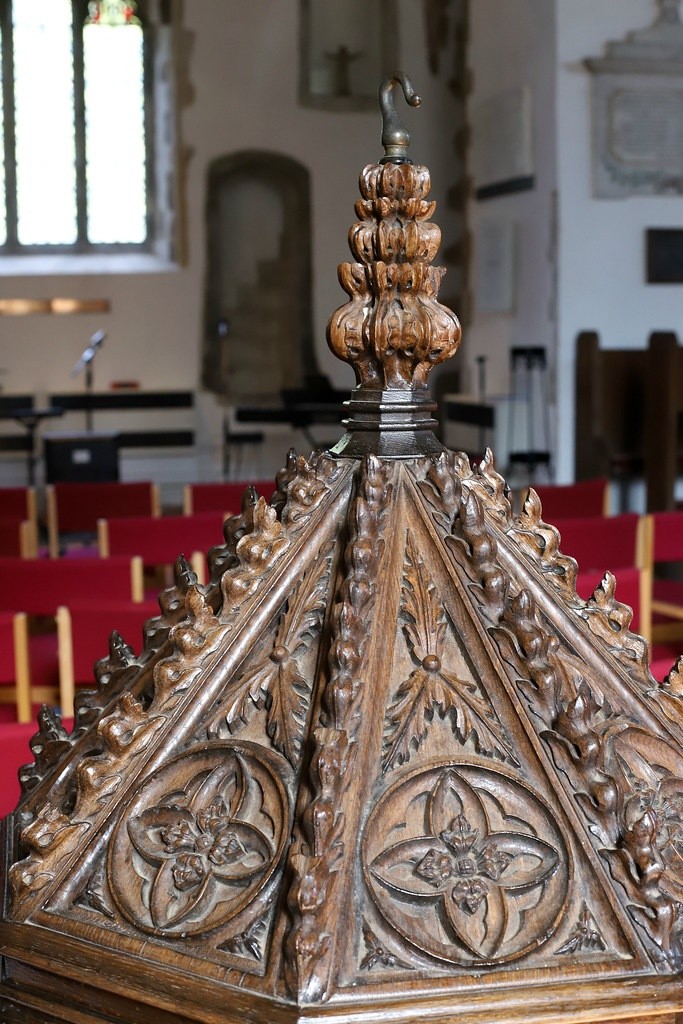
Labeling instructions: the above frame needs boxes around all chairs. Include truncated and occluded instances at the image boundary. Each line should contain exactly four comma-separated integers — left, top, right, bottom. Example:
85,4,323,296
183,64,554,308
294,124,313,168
0,471,682,822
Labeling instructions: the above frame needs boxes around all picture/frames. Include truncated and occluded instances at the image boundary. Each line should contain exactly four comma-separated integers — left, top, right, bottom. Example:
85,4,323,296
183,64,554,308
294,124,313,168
294,0,401,117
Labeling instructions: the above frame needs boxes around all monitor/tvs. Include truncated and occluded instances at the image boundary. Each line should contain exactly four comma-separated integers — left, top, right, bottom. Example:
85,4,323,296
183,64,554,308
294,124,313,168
42,434,123,484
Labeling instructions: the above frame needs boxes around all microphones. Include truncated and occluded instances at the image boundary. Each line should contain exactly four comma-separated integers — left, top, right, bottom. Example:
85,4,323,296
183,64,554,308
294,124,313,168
91,330,105,345
